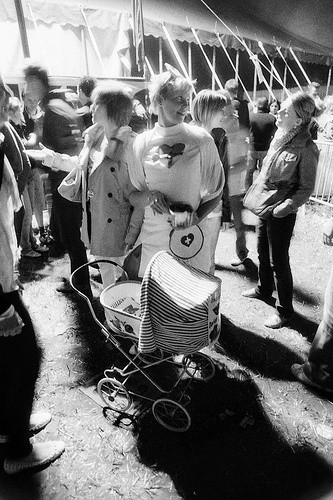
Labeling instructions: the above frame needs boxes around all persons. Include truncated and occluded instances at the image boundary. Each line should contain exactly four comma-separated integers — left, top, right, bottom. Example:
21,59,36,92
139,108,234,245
1,54,333,475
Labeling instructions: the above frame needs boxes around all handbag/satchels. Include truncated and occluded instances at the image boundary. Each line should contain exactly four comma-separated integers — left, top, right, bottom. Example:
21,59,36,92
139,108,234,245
242,176,291,220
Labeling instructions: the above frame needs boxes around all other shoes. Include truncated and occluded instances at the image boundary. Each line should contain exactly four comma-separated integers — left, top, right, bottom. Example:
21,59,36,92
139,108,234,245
0,412,52,443
21,250,41,257
3,441,66,475
35,246,50,252
242,288,272,300
231,256,248,265
291,363,333,392
264,310,296,329
57,284,77,293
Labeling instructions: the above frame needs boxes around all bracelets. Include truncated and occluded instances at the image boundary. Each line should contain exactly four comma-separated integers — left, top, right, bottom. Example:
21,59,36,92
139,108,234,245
148,190,160,201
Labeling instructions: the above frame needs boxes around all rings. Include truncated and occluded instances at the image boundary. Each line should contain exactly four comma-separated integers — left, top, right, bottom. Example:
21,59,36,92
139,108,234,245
154,198,158,203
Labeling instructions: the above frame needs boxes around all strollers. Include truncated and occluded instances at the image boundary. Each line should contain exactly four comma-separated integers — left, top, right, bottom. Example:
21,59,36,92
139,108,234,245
68,251,224,431
